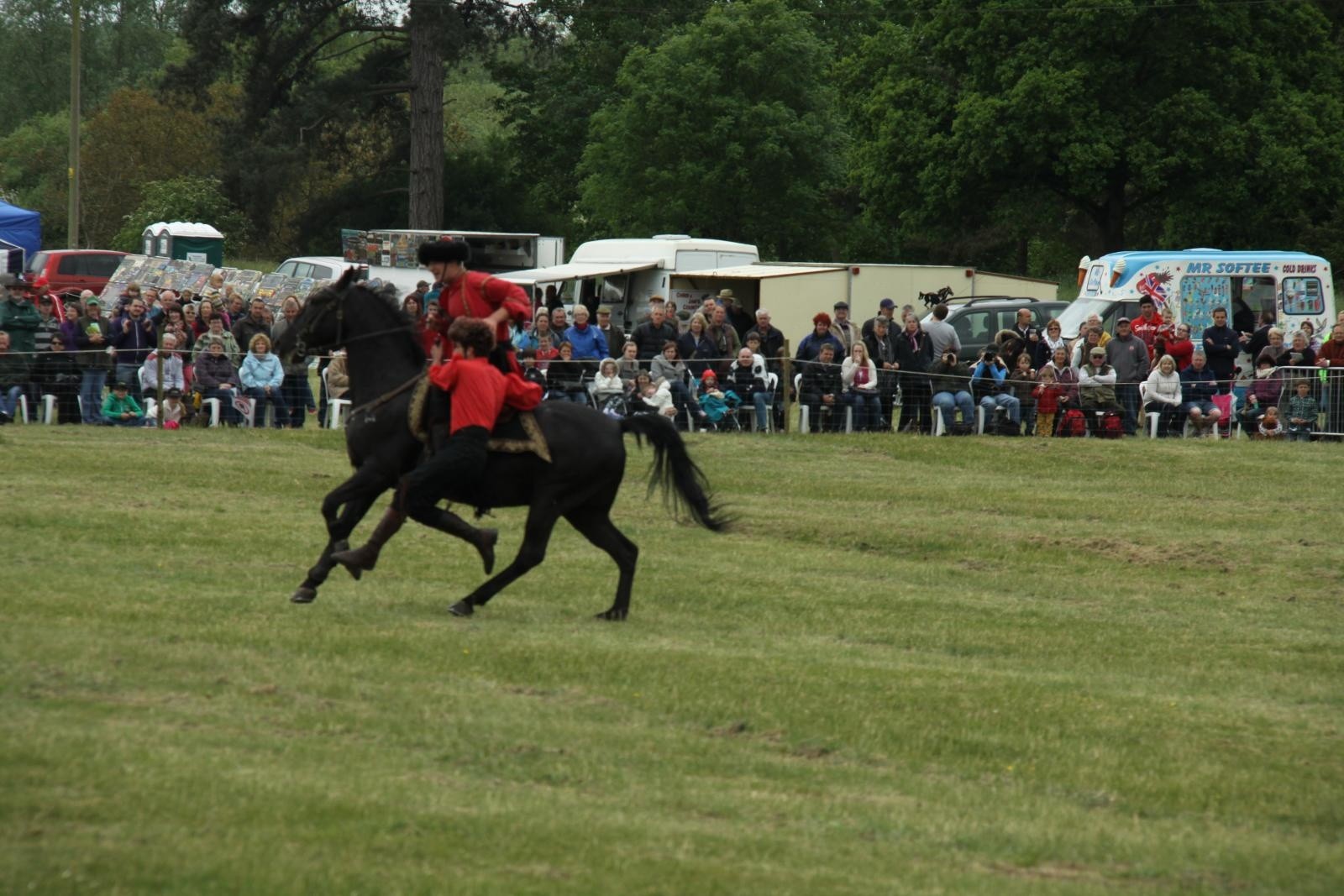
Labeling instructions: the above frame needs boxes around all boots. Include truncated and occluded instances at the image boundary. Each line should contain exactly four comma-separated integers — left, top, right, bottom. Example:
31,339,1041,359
437,509,498,576
328,506,406,571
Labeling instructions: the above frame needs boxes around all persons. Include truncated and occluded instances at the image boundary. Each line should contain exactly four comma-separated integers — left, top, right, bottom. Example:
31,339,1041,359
504,288,1344,442
418,240,542,457
0,274,445,428
331,315,542,571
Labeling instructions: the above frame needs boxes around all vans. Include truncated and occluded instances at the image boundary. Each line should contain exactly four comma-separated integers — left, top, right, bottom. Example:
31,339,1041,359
1038,248,1336,369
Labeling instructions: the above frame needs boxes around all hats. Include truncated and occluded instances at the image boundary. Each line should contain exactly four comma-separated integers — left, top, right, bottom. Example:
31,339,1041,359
596,307,611,314
113,381,129,390
1090,347,1106,355
881,298,898,310
702,370,716,380
716,289,736,300
86,296,99,305
834,302,849,309
650,295,664,302
1118,317,1130,326
419,234,471,264
167,388,182,397
4,279,31,288
39,295,53,303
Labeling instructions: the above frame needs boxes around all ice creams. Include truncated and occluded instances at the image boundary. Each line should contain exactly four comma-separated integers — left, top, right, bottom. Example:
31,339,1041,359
1076,255,1091,286
1040,383,1044,395
1030,369,1035,374
1109,259,1126,287
1287,280,1296,304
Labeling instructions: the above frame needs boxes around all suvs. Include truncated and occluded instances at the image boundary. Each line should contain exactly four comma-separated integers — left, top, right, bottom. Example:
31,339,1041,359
275,254,437,306
901,293,1071,362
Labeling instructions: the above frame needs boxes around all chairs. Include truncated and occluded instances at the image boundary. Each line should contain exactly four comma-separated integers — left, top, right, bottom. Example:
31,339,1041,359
41,393,86,425
138,367,161,426
1182,399,1221,441
953,318,973,339
193,365,223,427
1139,380,1178,439
740,372,778,435
0,392,29,427
969,379,1012,435
1238,385,1285,440
322,367,352,430
794,372,855,435
244,393,277,429
927,377,975,435
978,316,993,342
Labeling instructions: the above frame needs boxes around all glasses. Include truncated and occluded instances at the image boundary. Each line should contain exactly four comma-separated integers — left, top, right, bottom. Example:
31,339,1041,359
1092,355,1104,359
1049,328,1059,331
185,312,195,314
51,342,64,346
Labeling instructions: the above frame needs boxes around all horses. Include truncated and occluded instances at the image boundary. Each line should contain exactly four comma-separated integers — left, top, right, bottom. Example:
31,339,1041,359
274,266,740,621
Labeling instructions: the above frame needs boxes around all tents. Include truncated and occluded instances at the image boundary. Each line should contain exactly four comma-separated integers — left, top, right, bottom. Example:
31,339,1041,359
0,201,41,272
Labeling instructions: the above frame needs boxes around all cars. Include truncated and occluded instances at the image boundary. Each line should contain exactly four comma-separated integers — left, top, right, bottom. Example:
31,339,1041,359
18,249,135,309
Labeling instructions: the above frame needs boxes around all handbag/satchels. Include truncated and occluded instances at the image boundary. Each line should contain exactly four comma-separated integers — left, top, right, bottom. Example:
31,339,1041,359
853,385,880,396
998,417,1021,436
788,403,811,433
947,420,974,435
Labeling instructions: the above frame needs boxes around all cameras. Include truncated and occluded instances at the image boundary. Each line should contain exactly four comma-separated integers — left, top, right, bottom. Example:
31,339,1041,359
947,354,954,361
984,352,993,361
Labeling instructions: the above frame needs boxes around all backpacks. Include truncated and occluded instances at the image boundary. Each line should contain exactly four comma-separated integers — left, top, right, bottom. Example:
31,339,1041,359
1056,408,1086,437
1099,411,1123,439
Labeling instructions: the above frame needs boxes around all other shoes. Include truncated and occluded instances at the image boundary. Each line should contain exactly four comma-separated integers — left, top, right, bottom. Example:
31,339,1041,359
309,409,317,415
